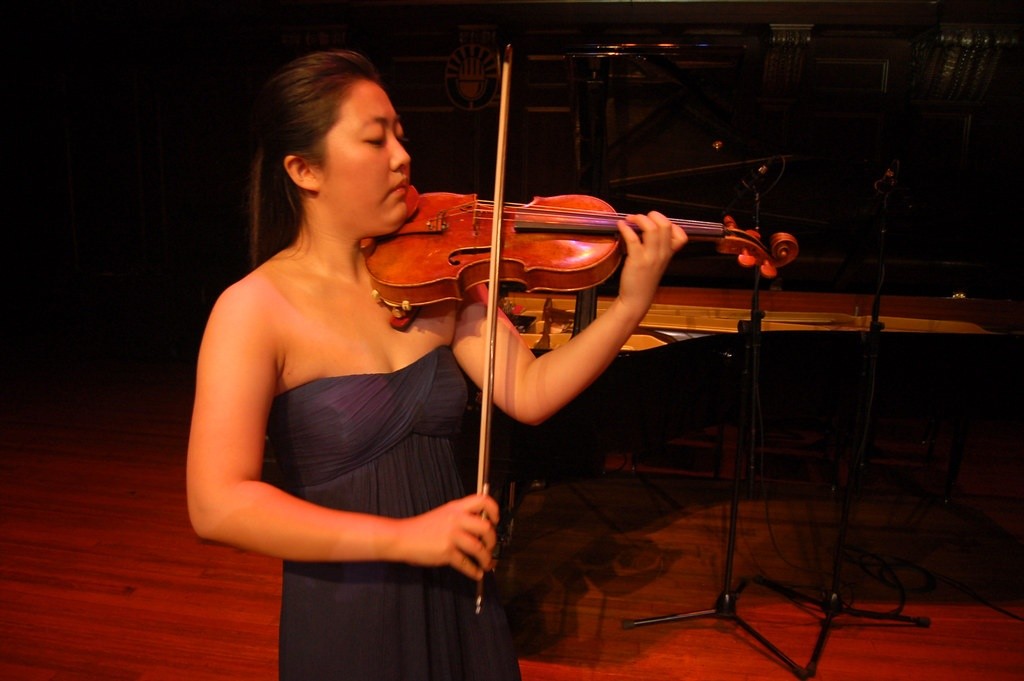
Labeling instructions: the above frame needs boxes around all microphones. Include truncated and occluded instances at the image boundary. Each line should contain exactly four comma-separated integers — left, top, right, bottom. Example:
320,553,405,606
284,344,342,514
873,167,895,195
743,164,767,192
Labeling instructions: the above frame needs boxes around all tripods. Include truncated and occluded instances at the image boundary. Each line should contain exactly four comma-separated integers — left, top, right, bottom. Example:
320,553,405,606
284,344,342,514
756,196,929,676
622,192,808,681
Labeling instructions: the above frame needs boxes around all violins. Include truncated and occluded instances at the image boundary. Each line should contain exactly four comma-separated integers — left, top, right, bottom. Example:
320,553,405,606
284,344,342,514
359,191,801,310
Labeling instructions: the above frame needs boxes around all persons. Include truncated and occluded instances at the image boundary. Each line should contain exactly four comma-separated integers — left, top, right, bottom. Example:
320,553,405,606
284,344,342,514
185,50,688,681
